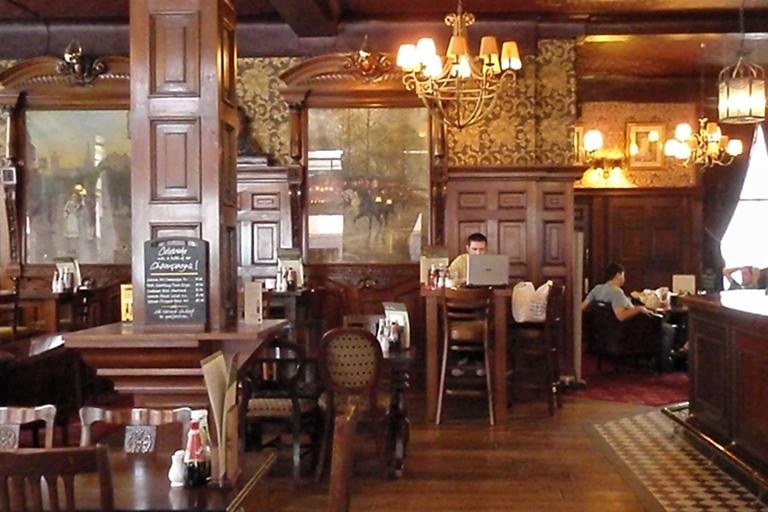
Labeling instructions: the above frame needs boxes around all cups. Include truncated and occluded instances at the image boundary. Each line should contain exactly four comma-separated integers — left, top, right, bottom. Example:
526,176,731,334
669,294,683,309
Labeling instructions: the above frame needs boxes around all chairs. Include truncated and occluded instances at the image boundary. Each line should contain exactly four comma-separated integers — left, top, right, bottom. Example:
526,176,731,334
343,314,386,336
436,285,497,427
507,282,564,417
582,260,767,387
242,338,315,480
1,443,113,511
314,327,396,481
77,406,191,452
0,262,309,372
0,405,56,450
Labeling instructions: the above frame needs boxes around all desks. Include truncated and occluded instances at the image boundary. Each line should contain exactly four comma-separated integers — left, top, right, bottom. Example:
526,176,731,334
258,328,417,462
2,449,277,511
420,283,512,421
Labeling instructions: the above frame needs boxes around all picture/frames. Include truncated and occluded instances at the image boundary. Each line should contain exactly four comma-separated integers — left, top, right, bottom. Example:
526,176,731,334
575,126,584,165
625,122,667,171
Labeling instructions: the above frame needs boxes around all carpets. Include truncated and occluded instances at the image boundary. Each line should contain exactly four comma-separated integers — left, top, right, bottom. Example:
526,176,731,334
583,404,767,511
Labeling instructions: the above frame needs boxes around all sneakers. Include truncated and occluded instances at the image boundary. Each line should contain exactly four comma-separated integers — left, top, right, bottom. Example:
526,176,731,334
451,357,469,377
473,360,486,377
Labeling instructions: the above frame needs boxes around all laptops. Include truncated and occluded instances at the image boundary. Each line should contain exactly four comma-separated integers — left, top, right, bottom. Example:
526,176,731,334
672,275,696,295
466,254,509,287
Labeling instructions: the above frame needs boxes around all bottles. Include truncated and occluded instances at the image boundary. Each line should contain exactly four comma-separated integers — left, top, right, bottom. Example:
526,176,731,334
181,420,212,488
443,266,450,278
166,450,188,490
375,319,401,358
427,265,438,291
275,266,294,293
49,267,71,294
436,264,443,289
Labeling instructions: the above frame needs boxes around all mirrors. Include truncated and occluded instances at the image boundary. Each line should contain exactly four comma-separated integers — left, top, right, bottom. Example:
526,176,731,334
0,38,134,271
274,30,453,268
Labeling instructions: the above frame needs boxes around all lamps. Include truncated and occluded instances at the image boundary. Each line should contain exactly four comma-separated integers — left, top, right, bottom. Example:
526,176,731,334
644,42,747,174
391,0,523,136
711,0,768,128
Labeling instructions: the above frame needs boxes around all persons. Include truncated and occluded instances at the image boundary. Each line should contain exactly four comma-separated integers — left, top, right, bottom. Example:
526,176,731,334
28,189,99,254
443,233,491,378
678,265,761,356
342,171,390,209
581,263,674,373
